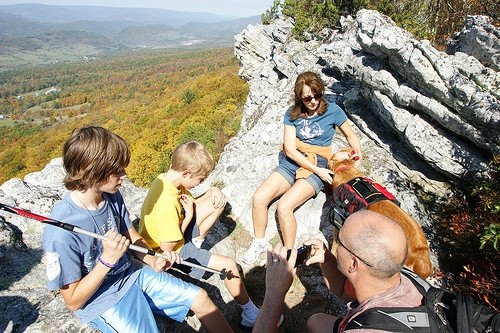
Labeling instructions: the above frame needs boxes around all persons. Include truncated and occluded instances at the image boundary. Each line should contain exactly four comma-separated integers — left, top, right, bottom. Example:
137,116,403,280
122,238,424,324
253,210,455,333
241,72,363,265
41,126,236,333
140,141,283,328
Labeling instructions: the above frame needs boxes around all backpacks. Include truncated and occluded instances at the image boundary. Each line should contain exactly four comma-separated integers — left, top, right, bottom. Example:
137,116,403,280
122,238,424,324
329,176,402,229
343,267,500,333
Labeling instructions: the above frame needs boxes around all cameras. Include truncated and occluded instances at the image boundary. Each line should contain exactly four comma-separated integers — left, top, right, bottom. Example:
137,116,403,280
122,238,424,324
287,244,313,268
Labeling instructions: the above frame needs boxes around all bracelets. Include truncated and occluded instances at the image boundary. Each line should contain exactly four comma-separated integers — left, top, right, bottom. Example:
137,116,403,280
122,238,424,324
99,257,119,268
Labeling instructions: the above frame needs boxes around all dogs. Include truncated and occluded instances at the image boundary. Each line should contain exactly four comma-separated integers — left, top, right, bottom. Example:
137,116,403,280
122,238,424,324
327,148,448,280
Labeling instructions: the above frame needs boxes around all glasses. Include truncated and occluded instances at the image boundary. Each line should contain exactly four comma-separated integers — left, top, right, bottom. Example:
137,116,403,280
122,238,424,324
300,92,323,102
335,231,373,268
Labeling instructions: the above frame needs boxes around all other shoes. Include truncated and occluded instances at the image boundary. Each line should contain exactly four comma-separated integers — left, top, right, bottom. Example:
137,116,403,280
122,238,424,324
240,311,285,332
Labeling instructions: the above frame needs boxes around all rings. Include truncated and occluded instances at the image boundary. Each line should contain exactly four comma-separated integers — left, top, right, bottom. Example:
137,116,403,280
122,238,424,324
272,259,280,264
